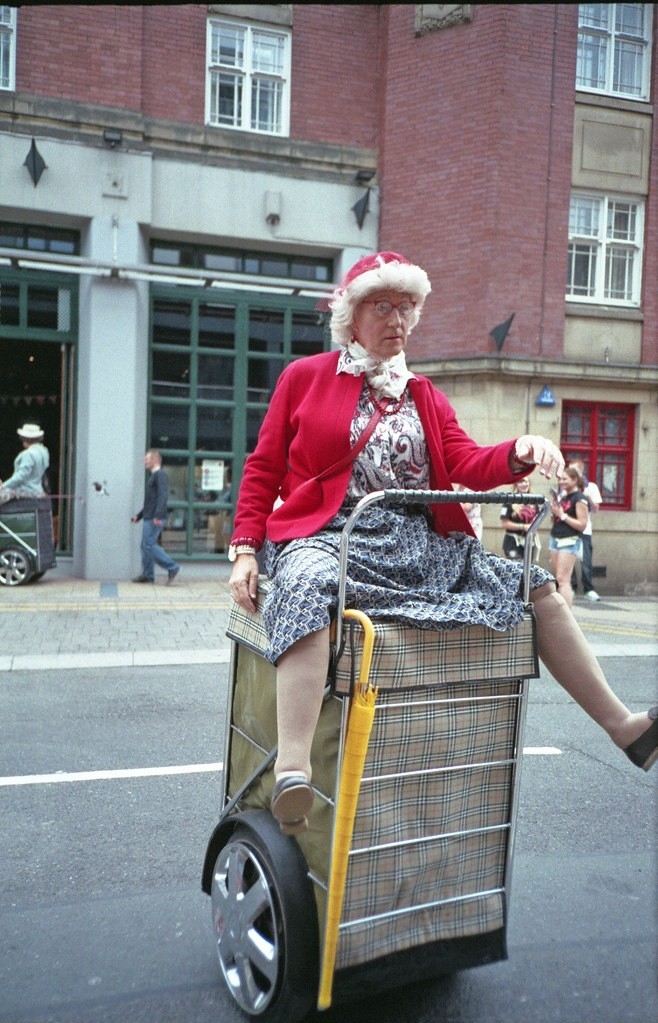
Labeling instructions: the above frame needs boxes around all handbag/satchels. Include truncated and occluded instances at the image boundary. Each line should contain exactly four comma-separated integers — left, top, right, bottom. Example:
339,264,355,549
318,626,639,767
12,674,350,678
40,466,59,495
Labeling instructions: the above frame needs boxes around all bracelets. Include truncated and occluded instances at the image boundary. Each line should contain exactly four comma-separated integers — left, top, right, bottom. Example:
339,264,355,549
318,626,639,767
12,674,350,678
512,440,533,467
518,524,523,531
228,537,262,562
560,513,568,521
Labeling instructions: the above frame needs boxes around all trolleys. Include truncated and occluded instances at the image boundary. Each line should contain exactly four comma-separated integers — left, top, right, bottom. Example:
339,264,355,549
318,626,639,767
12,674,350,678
201,486,552,1022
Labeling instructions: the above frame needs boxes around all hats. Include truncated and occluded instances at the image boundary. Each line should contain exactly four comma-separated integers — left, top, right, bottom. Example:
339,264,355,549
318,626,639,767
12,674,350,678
327,254,430,346
17,425,45,438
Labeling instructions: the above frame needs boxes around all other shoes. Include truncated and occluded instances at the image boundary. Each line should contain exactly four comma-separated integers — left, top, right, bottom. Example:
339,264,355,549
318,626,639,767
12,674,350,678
132,574,156,583
571,590,576,598
624,706,658,773
271,775,312,837
165,564,180,587
585,590,602,602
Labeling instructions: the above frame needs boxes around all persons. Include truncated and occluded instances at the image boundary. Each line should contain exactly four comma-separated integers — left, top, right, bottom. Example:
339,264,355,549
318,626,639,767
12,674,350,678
130,450,183,586
227,251,658,836
0,424,49,497
455,460,602,609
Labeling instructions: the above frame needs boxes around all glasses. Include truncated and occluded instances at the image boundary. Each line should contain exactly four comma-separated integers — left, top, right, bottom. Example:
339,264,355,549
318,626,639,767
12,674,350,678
359,301,416,318
